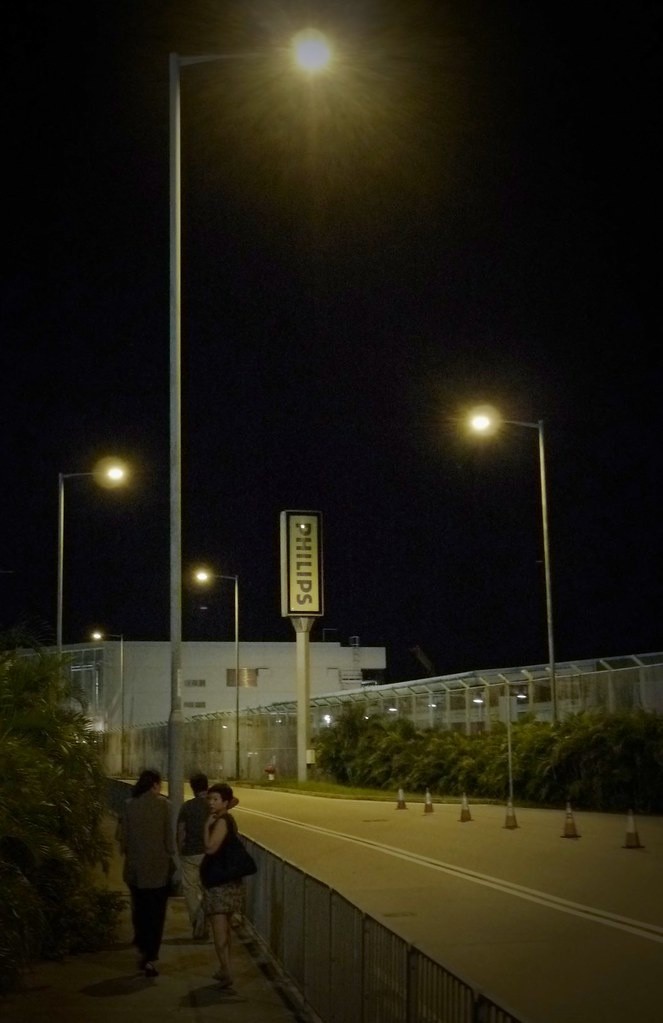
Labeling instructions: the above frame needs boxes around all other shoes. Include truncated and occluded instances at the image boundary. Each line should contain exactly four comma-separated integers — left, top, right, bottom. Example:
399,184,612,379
214,979,234,988
144,963,158,977
212,970,226,979
193,920,200,939
204,924,211,936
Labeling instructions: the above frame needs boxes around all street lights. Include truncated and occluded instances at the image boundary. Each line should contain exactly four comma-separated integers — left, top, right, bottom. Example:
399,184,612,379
92,632,128,778
196,568,243,782
468,404,563,731
164,21,345,802
57,458,127,651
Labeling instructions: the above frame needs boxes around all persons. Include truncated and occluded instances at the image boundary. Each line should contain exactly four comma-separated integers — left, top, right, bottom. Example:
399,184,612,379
114,770,178,976
199,784,244,990
176,773,239,941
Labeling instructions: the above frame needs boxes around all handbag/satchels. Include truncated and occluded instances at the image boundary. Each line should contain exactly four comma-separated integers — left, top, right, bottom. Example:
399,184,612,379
201,815,258,885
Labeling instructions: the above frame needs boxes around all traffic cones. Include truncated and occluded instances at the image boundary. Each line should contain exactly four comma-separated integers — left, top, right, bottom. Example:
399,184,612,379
502,797,521,829
457,791,474,823
621,808,647,850
394,788,409,810
560,802,583,839
422,787,434,813
